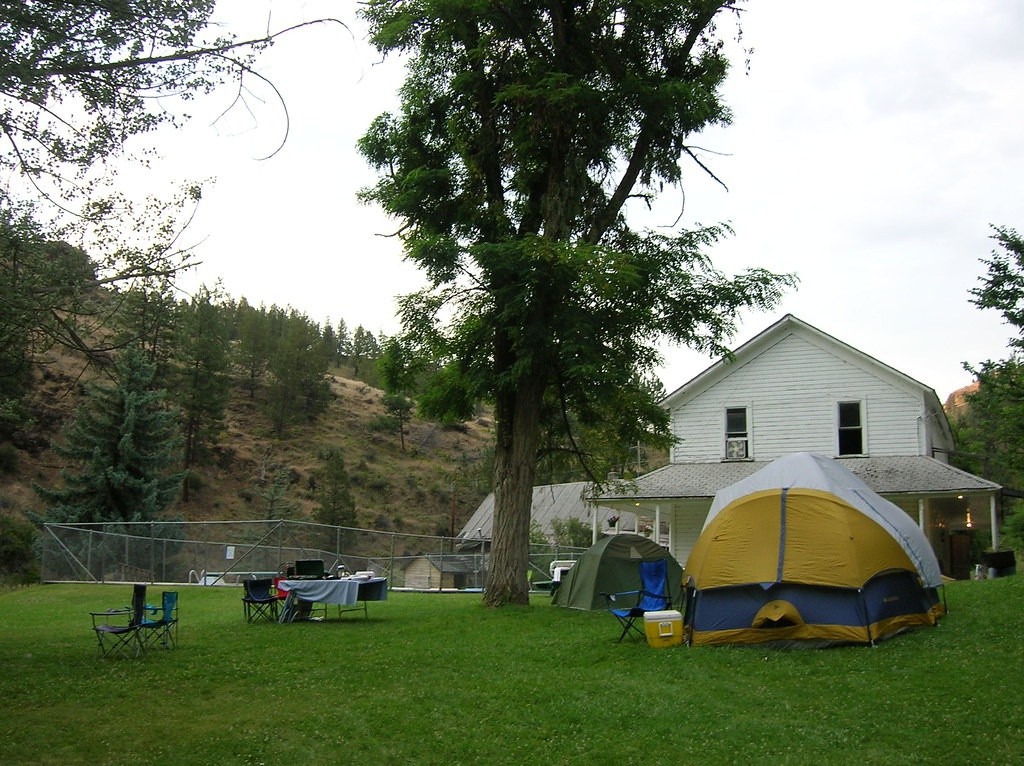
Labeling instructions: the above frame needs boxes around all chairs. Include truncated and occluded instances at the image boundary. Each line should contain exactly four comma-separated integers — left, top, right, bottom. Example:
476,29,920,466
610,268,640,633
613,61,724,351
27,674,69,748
599,558,675,644
89,584,179,659
241,576,289,624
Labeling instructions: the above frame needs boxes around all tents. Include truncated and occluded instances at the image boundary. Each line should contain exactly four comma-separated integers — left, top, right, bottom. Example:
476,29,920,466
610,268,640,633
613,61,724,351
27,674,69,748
682,452,944,647
552,534,684,612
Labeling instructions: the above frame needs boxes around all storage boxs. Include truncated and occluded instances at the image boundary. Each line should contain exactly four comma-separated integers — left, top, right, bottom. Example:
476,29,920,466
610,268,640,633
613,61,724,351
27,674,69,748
642,610,686,648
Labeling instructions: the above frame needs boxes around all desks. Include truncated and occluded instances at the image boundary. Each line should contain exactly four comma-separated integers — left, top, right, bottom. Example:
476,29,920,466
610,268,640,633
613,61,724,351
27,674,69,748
280,575,387,619
